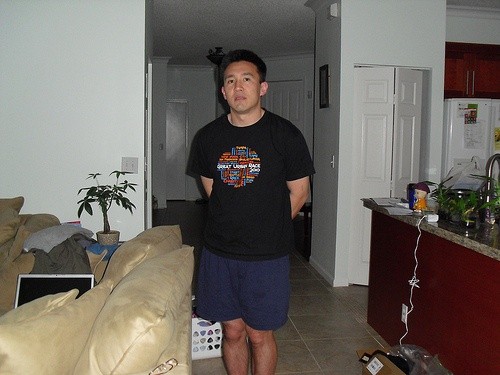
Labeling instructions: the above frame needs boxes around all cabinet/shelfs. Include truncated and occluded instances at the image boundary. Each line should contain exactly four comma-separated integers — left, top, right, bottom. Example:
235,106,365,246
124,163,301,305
443,41,500,100
351,65,429,285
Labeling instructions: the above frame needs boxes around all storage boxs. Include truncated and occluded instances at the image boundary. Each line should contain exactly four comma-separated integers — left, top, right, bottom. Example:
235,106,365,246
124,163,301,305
358,345,408,375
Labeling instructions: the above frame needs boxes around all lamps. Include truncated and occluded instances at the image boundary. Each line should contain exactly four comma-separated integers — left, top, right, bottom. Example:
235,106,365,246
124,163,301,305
207,46,225,65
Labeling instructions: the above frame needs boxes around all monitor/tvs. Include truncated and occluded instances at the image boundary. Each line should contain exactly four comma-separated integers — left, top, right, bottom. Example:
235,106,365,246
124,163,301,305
13,273,95,310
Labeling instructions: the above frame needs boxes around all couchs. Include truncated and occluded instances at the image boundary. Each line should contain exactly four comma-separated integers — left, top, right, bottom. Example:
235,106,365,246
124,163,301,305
0,195,199,374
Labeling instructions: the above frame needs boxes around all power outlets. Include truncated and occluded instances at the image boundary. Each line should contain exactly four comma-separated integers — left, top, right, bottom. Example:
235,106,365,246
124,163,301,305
402,305,409,322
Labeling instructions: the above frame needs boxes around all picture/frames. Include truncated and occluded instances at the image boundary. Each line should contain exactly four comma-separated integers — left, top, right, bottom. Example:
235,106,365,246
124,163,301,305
319,64,329,109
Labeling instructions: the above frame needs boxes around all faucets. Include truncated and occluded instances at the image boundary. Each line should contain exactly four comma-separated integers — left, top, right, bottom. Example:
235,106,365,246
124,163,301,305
485,153,500,223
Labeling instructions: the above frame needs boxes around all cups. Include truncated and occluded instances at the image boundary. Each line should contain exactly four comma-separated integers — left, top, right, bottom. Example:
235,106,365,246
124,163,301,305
408,184,418,210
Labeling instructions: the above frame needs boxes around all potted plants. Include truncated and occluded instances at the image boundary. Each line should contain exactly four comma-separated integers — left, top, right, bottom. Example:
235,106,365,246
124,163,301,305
428,170,500,233
77,170,137,246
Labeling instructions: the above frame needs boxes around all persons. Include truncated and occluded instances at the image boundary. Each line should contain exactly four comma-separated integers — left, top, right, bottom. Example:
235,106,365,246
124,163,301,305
186,49,316,375
413,181,430,212
